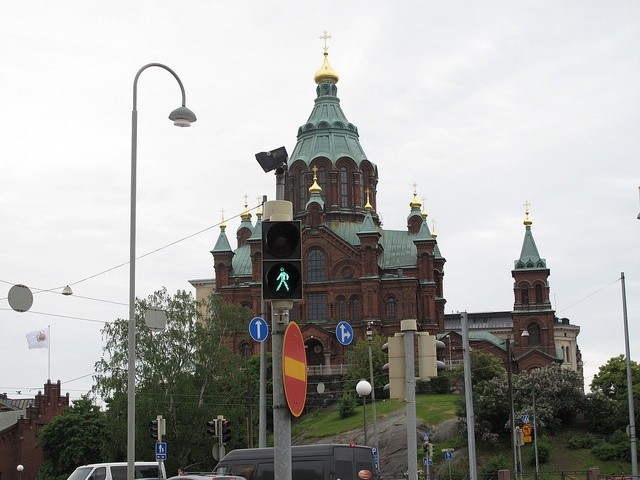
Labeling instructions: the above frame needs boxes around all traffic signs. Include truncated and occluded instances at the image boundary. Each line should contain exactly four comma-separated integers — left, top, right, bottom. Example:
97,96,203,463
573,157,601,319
282,320,307,417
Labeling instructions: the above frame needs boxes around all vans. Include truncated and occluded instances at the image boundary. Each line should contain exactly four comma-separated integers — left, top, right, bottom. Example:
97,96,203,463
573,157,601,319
210,443,376,479
66,461,166,480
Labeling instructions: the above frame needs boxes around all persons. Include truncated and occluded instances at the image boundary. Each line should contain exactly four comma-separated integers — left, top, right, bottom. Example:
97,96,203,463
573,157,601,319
177,467,188,476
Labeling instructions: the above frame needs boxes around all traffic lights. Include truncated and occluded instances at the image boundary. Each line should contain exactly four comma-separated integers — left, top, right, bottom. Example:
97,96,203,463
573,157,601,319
151,420,158,440
423,443,428,452
429,443,435,457
262,220,303,301
417,335,447,381
222,421,231,443
382,337,406,400
207,420,215,437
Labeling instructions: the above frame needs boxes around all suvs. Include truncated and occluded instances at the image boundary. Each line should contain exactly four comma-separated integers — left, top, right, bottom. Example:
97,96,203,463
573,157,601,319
165,472,246,480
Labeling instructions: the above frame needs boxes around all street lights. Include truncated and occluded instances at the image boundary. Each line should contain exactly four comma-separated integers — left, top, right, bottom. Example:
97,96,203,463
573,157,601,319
17,465,24,480
127,63,196,479
356,377,372,444
